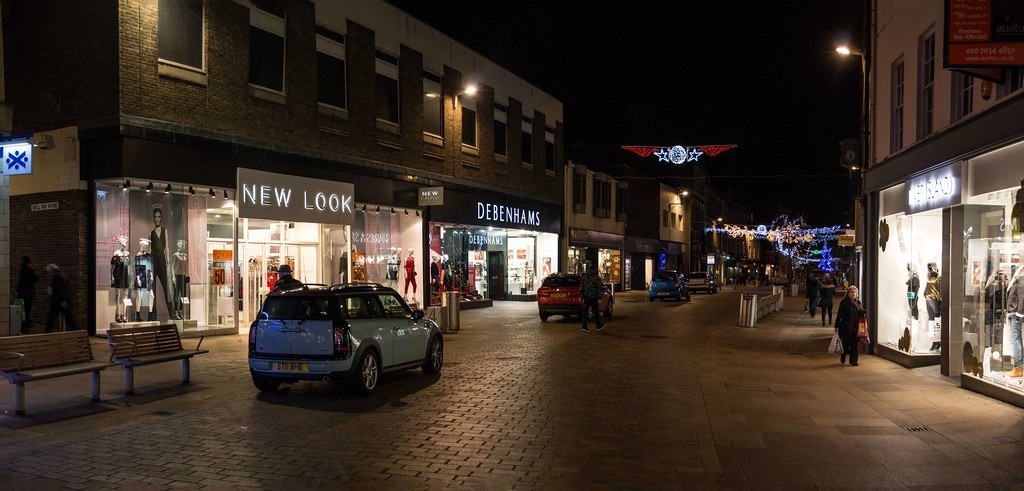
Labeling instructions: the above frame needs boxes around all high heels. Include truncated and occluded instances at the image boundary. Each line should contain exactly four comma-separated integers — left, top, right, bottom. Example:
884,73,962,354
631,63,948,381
120,314,127,323
412,298,417,302
115,313,122,323
404,297,409,302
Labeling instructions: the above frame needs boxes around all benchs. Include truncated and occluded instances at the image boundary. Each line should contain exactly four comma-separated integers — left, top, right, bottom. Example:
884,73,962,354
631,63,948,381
758,293,780,320
0,329,117,415
106,324,208,394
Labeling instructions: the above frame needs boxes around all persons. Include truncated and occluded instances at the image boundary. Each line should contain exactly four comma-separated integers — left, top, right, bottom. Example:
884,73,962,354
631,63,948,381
272,264,303,291
385,247,402,289
1006,263,1024,377
905,263,920,351
923,262,942,350
171,239,188,320
441,254,453,290
338,225,358,283
16,255,39,334
820,277,836,327
834,285,866,366
45,263,72,333
148,208,174,320
403,248,417,302
134,238,156,321
973,268,981,346
806,274,821,317
109,237,130,323
431,255,440,296
579,266,606,333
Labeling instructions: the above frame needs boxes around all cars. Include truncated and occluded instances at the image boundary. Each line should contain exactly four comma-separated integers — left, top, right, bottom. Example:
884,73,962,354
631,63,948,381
686,271,718,294
648,269,691,302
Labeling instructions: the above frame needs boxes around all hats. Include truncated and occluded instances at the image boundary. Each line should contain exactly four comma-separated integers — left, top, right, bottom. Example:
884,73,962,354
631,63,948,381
276,264,293,273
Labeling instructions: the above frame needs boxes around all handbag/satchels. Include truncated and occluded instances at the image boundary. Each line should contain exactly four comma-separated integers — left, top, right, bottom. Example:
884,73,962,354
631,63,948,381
12,298,25,321
828,331,844,355
856,312,870,344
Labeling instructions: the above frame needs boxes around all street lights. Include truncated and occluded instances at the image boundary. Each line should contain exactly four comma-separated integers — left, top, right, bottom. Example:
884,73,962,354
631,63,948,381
834,44,867,167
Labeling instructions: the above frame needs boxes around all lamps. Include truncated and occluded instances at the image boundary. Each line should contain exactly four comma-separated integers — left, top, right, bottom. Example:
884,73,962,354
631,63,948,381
123,180,228,199
354,203,420,217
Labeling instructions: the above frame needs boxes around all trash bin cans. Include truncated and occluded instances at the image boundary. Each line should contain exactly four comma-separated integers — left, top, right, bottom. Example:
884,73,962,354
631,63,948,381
773,282,786,314
792,285,798,298
739,293,758,328
442,292,461,331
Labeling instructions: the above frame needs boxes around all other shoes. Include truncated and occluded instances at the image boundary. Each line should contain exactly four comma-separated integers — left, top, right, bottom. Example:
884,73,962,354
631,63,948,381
581,327,590,333
596,322,605,331
1006,367,1023,377
842,361,845,366
930,340,941,350
850,361,859,366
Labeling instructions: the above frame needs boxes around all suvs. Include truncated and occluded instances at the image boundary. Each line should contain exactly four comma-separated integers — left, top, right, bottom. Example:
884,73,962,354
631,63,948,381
246,281,446,397
537,272,614,323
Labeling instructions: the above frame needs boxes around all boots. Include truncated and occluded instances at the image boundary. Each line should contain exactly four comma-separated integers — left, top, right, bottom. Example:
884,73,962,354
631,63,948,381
178,310,183,319
147,312,155,321
173,310,181,320
136,312,143,322
